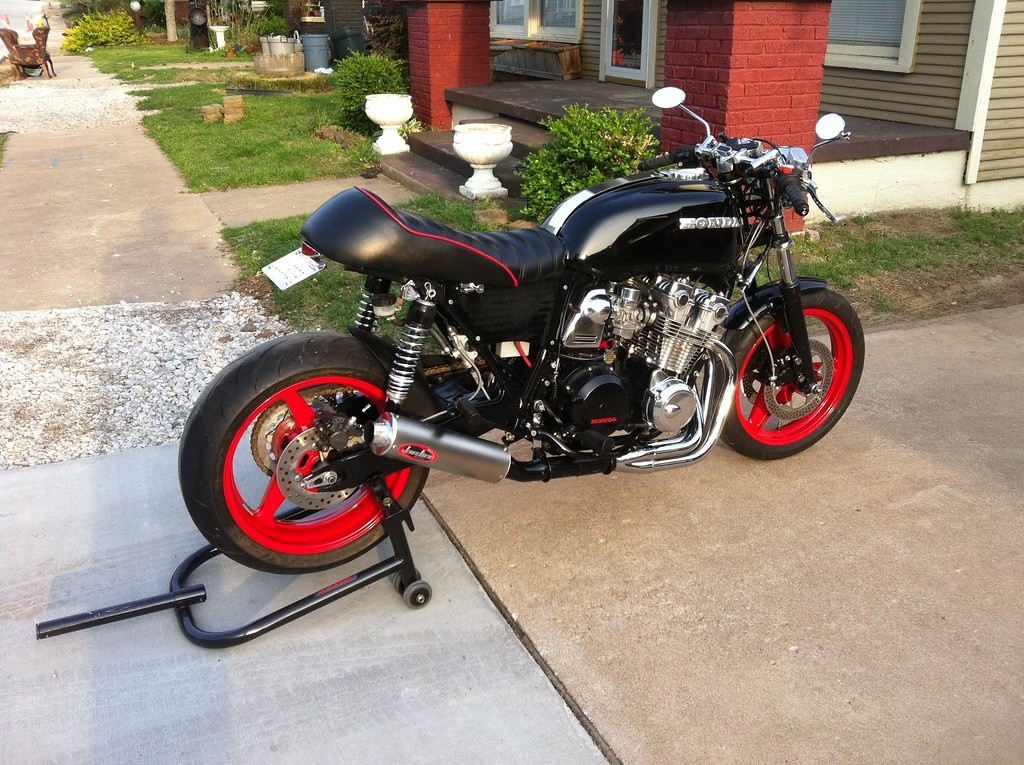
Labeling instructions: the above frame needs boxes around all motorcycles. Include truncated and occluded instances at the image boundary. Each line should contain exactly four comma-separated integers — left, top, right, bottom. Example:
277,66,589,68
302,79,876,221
177,86,865,575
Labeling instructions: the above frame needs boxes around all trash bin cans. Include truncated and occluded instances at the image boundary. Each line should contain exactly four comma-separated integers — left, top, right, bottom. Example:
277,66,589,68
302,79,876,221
259,34,296,57
330,25,365,60
300,32,330,72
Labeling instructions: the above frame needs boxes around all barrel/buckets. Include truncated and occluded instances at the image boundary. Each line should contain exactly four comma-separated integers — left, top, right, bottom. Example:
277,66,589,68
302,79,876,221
268,37,296,54
300,34,329,71
260,37,271,56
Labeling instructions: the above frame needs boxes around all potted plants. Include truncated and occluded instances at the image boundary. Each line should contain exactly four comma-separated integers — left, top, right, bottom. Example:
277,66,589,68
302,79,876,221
267,17,295,56
300,2,313,17
251,18,271,56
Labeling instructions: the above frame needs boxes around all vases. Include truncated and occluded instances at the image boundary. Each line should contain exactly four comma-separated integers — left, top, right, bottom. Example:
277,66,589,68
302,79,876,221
364,94,413,155
453,123,513,201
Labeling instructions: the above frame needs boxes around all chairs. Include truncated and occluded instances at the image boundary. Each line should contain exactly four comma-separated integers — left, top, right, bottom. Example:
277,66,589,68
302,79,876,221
21,26,57,77
0,28,52,80
